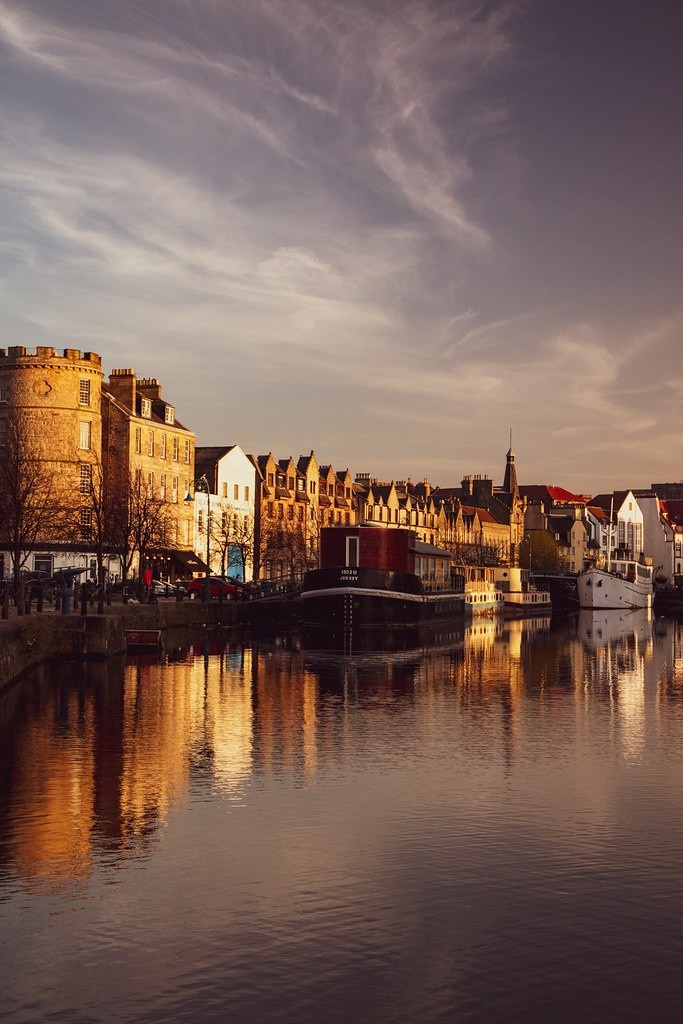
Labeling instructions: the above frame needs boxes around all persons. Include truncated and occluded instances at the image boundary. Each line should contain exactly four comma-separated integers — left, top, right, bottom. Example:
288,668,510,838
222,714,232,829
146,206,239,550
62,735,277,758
236,574,239,579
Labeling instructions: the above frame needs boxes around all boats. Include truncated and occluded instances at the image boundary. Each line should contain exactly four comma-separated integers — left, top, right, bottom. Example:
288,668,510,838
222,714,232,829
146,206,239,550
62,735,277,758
576,495,657,611
297,523,469,628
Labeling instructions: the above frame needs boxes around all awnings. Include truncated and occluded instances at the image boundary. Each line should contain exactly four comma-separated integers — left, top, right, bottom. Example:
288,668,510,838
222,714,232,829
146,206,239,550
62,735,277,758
263,484,270,499
319,496,332,506
174,551,213,572
296,492,310,503
275,487,292,500
351,499,358,509
335,497,349,508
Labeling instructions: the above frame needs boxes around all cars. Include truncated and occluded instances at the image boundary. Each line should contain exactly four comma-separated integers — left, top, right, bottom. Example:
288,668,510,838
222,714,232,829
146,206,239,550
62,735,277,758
153,579,191,597
259,579,276,592
187,575,244,601
